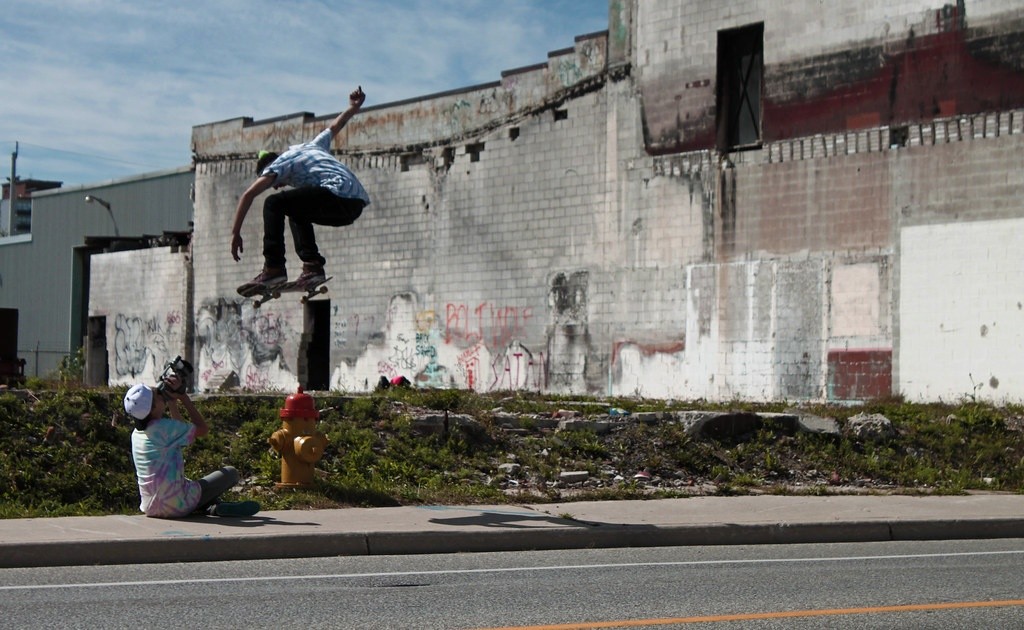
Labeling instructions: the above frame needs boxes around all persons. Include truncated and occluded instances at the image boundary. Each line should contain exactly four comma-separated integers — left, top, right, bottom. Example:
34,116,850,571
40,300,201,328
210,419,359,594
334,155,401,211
123,373,260,517
230,85,371,285
378,375,412,391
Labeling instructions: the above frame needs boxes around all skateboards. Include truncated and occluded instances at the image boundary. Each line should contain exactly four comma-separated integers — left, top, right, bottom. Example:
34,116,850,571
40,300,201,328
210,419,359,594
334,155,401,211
236,275,333,309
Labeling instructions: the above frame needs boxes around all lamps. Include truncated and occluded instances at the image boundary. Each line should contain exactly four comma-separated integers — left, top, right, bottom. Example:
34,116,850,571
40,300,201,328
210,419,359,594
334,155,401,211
84,195,111,210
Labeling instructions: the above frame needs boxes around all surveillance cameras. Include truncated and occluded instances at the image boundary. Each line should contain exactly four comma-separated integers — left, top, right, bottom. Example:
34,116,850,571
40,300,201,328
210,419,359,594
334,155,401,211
85,195,92,202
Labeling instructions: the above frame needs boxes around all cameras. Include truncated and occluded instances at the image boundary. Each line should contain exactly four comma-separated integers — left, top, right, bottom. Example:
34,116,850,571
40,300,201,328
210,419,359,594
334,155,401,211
155,355,194,401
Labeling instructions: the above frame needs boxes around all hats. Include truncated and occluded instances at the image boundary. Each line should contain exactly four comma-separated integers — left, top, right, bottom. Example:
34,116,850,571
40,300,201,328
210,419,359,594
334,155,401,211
123,386,154,423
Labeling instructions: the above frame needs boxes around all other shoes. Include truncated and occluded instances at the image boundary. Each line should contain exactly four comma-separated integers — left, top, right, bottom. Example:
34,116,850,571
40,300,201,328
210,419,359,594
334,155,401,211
300,258,326,282
253,265,287,283
215,500,260,518
